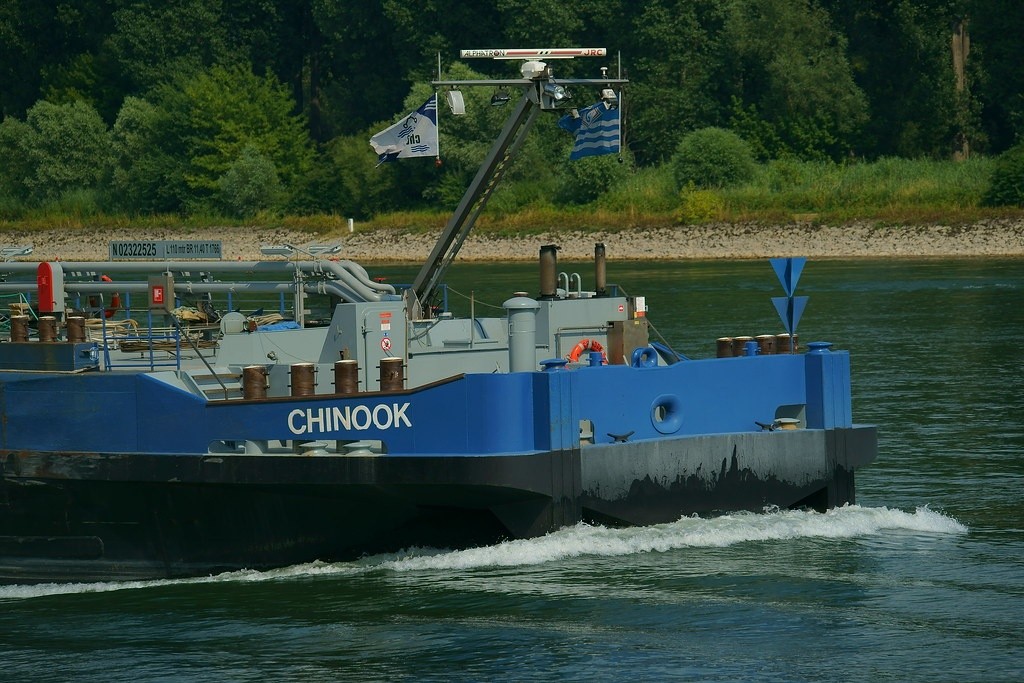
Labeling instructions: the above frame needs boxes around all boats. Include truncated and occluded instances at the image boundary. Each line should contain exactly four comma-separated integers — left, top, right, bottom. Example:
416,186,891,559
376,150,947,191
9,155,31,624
261,245,293,255
0,47,879,556
309,246,341,254
0,247,32,255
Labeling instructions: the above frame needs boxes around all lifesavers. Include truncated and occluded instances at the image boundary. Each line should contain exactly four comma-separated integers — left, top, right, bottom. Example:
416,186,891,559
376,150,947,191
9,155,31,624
89,275,119,318
569,338,609,366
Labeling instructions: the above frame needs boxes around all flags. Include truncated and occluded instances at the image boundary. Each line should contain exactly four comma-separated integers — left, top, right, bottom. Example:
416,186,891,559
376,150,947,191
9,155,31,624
370,95,437,168
558,102,619,161
